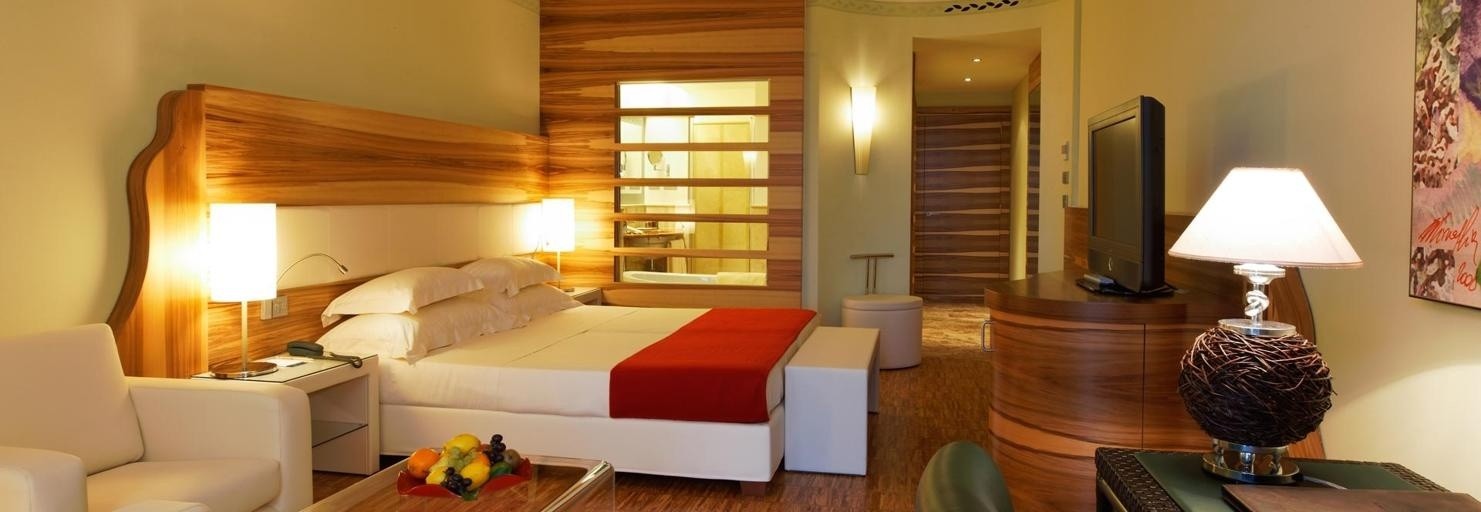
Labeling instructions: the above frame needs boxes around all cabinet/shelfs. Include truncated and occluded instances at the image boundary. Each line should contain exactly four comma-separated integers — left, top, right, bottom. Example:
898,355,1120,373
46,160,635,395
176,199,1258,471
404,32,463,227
191,348,380,476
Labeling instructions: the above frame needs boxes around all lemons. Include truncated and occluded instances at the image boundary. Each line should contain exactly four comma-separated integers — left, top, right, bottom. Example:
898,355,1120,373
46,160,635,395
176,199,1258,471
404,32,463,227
460,462,490,491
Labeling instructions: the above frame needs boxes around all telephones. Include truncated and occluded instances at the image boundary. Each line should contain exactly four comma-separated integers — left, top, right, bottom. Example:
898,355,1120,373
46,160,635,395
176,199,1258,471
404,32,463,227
288,341,323,357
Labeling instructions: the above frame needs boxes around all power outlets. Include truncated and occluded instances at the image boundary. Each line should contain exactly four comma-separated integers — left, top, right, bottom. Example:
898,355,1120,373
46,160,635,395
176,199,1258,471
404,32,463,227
273,296,288,318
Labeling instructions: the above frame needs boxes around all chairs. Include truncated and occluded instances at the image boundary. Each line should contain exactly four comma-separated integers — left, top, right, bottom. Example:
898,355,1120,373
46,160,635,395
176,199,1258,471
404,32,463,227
842,252,923,371
0,321,314,512
916,441,1015,511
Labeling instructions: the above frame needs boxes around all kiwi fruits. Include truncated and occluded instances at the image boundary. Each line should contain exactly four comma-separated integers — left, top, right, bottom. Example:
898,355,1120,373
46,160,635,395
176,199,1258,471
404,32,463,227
502,449,521,467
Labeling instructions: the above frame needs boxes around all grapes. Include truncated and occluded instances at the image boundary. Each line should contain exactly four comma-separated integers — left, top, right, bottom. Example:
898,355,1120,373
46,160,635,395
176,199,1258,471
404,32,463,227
440,467,472,493
482,434,505,464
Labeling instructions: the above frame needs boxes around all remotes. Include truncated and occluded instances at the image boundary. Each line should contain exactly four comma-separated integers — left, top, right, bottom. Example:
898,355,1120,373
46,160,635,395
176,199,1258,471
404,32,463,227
1083,271,1114,286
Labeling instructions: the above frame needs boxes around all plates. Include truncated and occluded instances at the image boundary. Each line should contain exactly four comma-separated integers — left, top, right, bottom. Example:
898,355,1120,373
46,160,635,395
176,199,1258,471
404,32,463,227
397,458,535,500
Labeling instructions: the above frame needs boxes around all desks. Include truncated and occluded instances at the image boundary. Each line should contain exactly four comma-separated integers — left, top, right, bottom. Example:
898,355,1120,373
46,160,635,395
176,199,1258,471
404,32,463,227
565,286,603,307
1095,448,1478,512
986,210,1325,512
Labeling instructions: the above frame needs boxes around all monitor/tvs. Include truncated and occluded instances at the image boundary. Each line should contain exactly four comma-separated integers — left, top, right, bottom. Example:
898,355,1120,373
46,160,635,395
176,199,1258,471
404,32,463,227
1076,95,1175,297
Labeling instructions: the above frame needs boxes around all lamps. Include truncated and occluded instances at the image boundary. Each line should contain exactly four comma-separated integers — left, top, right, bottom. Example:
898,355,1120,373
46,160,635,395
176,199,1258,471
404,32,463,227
1168,165,1362,482
850,86,877,177
279,252,348,283
543,199,575,291
207,202,279,379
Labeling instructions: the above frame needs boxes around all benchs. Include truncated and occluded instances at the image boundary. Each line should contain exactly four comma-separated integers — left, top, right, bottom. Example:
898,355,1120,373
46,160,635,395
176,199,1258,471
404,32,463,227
785,326,881,476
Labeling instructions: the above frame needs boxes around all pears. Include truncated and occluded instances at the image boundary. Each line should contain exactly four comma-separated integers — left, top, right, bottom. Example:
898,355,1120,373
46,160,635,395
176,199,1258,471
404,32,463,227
427,457,447,471
424,466,449,484
443,433,480,454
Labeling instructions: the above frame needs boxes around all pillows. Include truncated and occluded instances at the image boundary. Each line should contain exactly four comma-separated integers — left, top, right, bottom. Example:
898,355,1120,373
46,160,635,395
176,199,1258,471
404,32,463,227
479,282,585,320
314,294,524,367
321,267,485,328
458,256,565,299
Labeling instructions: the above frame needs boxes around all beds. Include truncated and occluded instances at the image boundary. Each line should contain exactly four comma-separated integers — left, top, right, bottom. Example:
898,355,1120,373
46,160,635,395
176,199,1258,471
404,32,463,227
314,305,821,497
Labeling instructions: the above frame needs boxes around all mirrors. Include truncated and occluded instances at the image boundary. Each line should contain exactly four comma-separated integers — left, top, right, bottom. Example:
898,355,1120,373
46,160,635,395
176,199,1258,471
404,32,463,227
619,116,647,206
